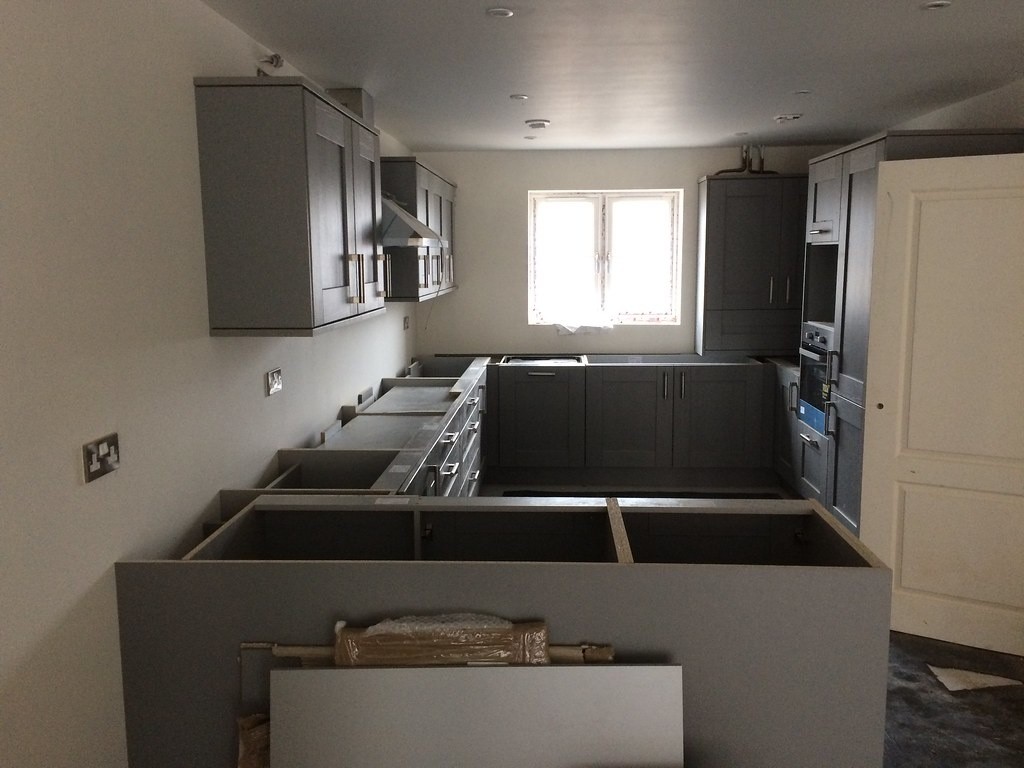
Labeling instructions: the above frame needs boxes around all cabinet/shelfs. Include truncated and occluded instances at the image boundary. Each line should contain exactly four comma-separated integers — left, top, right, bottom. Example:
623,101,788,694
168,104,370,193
584,354,774,488
218,413,464,498
798,127,1024,539
694,173,808,357
382,157,459,303
341,356,491,498
497,353,583,488
766,358,799,487
193,77,387,337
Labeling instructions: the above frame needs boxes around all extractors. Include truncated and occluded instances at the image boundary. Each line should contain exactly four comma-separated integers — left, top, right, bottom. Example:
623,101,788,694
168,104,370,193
381,191,448,249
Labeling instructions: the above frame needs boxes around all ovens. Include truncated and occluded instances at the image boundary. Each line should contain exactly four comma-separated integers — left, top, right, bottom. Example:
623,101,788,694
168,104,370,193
798,322,834,436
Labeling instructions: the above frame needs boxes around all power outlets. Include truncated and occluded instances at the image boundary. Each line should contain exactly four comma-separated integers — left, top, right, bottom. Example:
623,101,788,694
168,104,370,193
267,368,283,395
81,431,122,484
403,316,409,330
